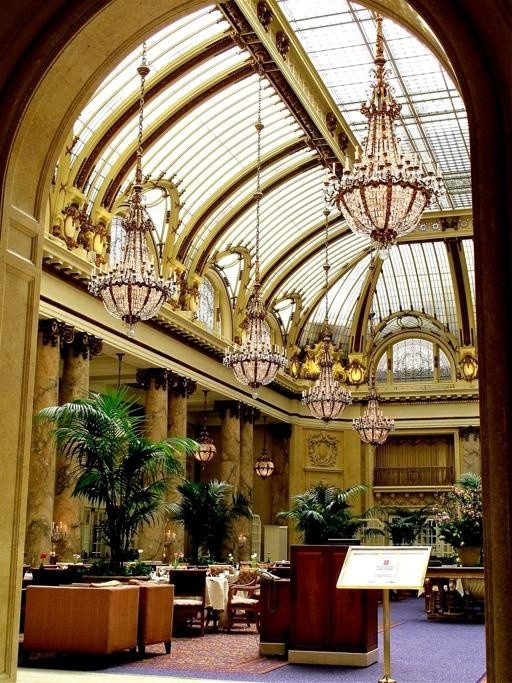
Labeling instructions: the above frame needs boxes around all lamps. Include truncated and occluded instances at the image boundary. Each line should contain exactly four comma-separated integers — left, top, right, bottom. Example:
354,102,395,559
301,185,353,429
90,34,178,337
188,387,217,473
51,521,69,541
350,253,396,450
237,533,249,544
323,1,440,252
255,414,276,481
223,60,293,401
162,528,179,545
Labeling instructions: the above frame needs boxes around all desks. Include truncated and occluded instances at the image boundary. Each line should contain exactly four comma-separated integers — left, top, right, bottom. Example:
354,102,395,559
424,565,484,622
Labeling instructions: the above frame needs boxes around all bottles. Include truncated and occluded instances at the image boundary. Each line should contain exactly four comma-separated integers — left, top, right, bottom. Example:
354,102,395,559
250,556,258,568
267,552,272,563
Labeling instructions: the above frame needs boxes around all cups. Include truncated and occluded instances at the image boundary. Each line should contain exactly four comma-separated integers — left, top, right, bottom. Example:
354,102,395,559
205,568,210,576
236,562,240,570
150,571,156,579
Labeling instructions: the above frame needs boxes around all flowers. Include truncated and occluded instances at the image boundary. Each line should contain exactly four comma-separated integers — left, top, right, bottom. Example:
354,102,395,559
425,473,484,546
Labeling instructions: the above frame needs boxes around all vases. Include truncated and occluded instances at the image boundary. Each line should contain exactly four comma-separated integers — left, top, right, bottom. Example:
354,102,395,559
453,544,482,568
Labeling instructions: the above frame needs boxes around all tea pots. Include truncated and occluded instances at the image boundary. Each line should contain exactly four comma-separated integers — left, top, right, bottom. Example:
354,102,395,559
156,565,170,581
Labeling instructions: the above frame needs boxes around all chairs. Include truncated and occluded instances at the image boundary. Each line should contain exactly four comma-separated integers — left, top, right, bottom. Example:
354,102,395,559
20,548,289,673
